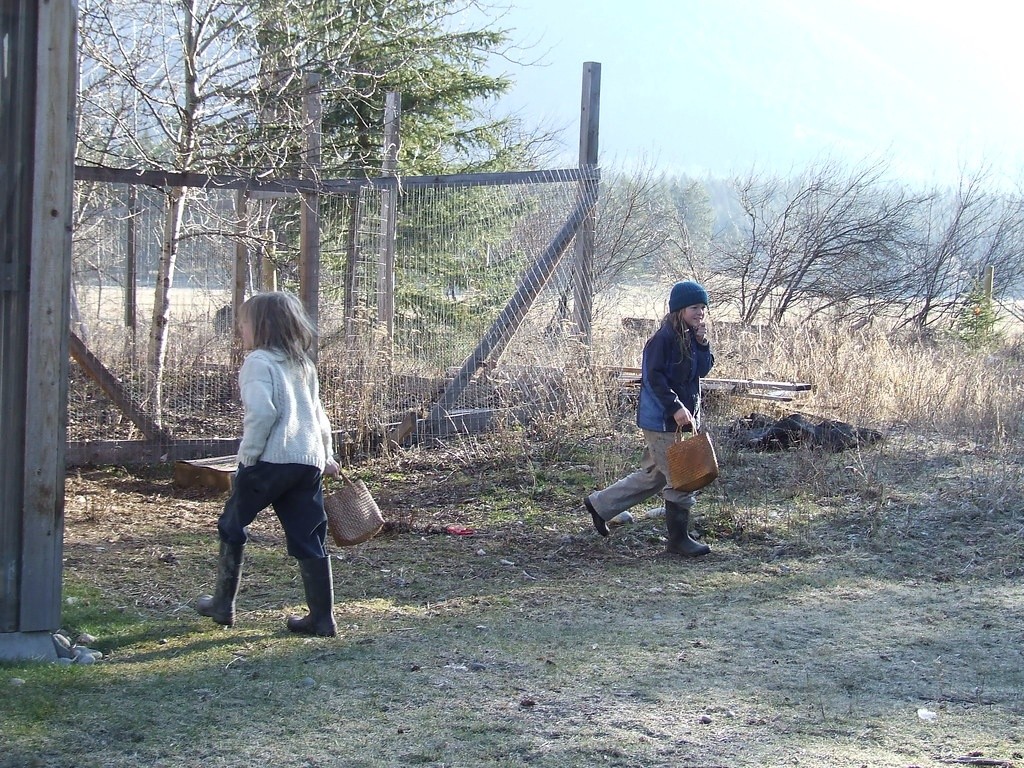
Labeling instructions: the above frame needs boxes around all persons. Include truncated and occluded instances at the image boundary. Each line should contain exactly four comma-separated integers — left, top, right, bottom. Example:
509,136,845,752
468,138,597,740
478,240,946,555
584,281,716,556
195,292,344,637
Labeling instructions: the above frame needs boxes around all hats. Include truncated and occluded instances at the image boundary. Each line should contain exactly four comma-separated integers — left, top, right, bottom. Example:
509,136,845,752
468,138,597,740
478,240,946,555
668,281,707,313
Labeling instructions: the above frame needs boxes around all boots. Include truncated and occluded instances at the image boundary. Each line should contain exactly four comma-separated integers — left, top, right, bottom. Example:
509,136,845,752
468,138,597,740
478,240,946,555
285,556,338,637
583,498,612,536
664,501,712,557
197,540,245,627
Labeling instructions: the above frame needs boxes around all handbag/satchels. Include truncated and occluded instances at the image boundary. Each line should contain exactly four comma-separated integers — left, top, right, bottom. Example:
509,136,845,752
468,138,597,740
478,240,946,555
666,420,719,492
323,470,386,547
